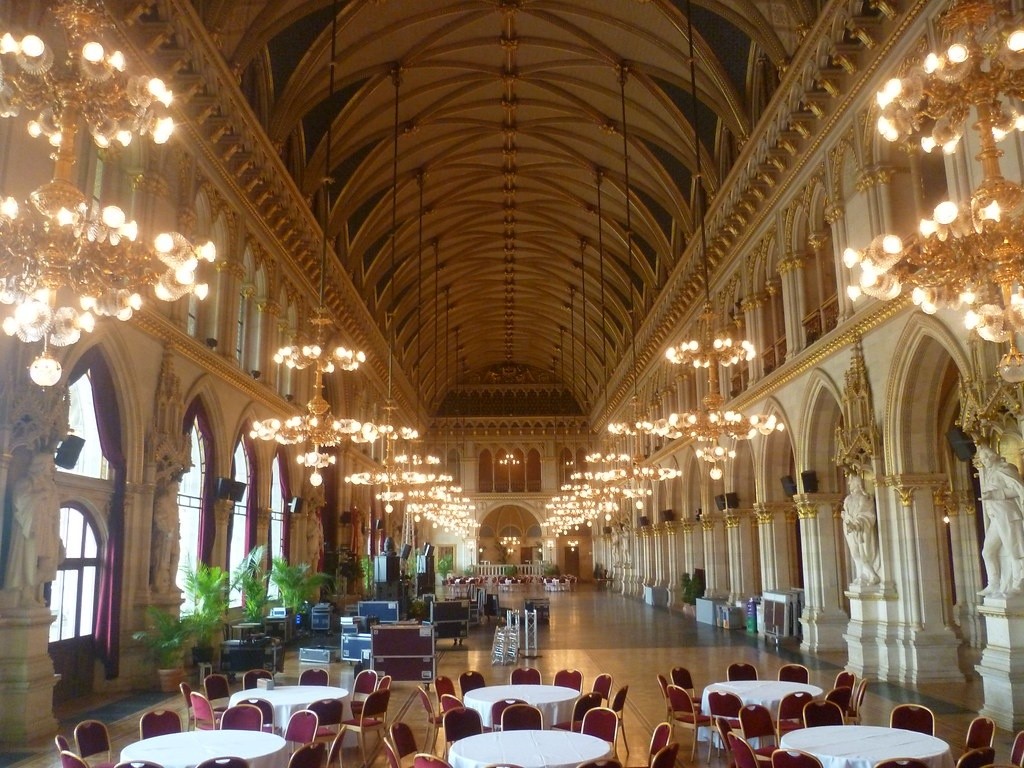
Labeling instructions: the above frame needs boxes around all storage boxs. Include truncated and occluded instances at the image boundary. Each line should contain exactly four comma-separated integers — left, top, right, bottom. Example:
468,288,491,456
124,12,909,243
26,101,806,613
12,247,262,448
220,595,549,673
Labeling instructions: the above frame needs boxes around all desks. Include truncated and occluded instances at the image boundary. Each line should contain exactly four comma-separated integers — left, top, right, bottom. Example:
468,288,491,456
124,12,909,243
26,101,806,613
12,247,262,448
444,731,615,768
696,680,824,750
227,686,354,738
779,726,957,768
120,730,289,768
464,684,580,728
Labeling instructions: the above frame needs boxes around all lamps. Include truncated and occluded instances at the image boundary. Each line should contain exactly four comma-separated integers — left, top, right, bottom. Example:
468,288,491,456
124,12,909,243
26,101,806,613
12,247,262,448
0,0,1022,543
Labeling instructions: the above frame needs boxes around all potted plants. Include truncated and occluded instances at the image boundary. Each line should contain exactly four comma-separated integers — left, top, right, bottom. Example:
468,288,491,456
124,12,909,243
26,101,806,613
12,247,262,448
131,604,208,694
184,559,231,667
439,555,454,585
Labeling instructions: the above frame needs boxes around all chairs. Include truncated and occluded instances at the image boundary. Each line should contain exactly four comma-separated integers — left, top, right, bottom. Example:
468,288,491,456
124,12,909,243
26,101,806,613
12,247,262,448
442,574,578,592
55,662,1024,768
